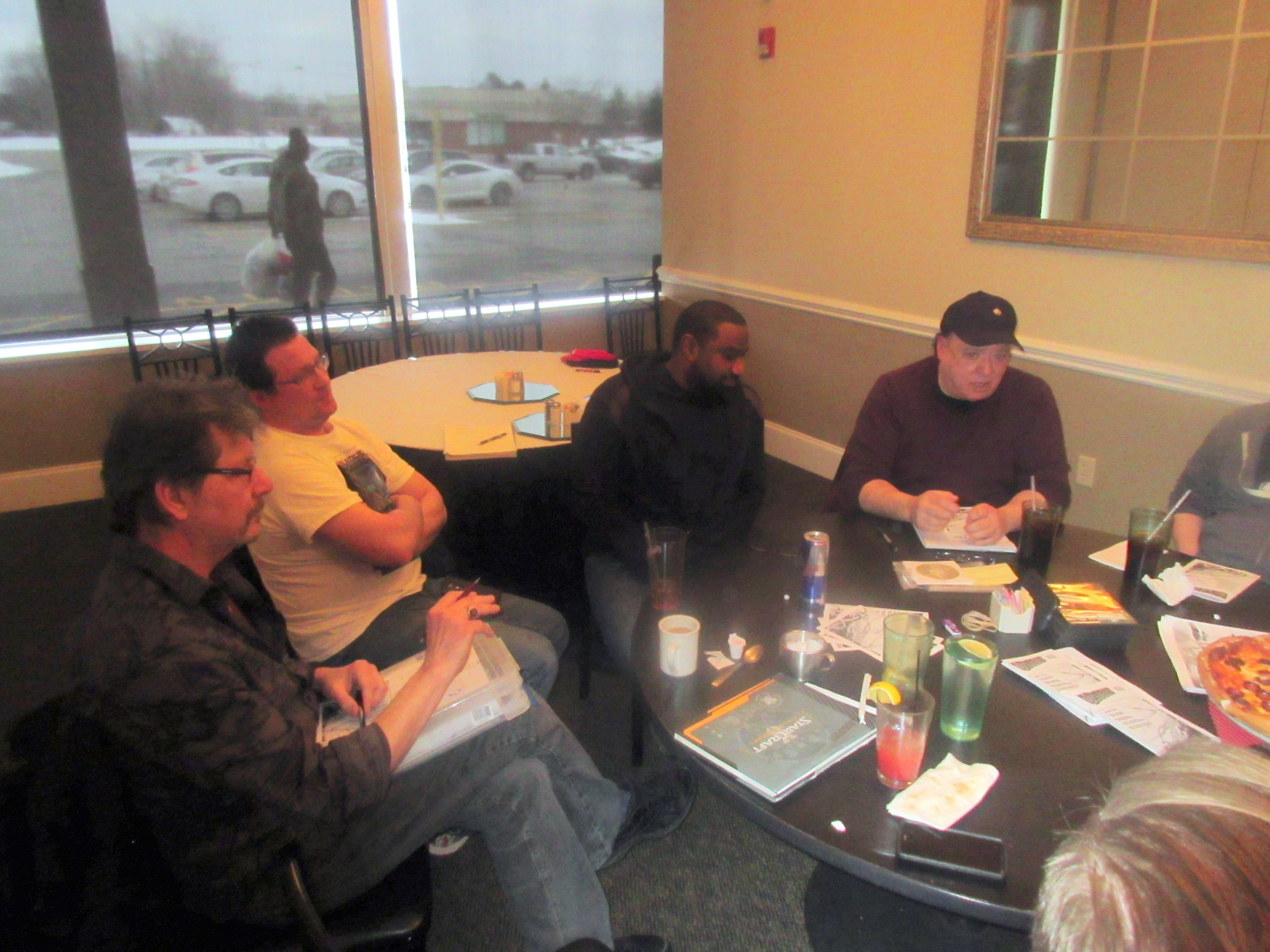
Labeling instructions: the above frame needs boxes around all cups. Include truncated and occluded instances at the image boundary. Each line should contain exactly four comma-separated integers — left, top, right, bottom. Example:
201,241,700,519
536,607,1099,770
1123,507,1175,584
1017,498,1063,573
777,629,836,683
882,612,935,688
939,633,1000,741
644,525,688,612
657,613,700,677
875,685,936,789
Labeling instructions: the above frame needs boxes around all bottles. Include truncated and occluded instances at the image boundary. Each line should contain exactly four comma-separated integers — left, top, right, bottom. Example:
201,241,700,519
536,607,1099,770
545,399,561,425
511,371,525,394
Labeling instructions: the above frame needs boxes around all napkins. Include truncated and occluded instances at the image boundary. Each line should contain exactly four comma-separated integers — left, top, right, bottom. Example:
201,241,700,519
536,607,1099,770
883,753,997,827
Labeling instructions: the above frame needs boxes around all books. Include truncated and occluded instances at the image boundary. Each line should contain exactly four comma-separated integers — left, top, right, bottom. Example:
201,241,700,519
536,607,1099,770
673,672,879,798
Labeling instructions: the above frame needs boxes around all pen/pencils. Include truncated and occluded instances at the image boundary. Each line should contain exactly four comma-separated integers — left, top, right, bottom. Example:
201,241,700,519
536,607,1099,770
877,527,892,544
576,369,603,373
454,577,480,603
480,433,506,445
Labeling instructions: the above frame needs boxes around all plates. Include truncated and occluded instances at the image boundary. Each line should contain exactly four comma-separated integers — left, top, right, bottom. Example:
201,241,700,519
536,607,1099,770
1206,691,1270,744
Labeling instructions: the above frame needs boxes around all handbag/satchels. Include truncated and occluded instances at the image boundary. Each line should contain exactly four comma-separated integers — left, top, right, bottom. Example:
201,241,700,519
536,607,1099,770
244,232,297,300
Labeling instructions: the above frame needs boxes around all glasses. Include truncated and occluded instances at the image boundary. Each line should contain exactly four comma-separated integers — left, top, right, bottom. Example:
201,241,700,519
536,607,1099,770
272,351,329,390
200,454,259,484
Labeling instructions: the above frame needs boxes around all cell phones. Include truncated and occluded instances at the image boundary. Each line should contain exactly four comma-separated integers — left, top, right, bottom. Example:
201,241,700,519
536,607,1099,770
895,820,1007,885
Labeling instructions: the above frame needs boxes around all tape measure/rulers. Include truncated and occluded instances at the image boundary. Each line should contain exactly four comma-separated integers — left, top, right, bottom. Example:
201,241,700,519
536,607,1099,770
961,610,998,633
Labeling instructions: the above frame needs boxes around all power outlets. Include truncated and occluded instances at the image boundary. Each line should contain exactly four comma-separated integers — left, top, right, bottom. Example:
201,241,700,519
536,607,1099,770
1075,452,1098,487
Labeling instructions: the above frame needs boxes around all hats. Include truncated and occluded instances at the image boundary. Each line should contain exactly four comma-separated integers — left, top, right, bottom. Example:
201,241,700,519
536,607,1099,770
940,291,1024,352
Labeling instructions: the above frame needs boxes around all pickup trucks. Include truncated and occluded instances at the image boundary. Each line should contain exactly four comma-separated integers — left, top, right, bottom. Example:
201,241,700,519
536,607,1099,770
502,143,600,182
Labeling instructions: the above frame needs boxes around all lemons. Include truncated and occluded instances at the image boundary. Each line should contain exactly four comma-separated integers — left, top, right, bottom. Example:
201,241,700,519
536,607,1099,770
865,682,901,706
882,668,909,682
957,638,993,659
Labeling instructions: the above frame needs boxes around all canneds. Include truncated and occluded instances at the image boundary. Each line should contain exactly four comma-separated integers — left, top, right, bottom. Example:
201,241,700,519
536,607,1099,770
799,600,823,633
799,530,830,603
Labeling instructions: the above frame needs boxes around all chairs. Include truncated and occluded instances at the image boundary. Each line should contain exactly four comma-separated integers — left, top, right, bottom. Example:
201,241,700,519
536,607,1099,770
400,288,477,356
474,282,543,352
319,294,402,377
604,276,662,361
0,437,651,950
228,303,315,350
122,308,222,382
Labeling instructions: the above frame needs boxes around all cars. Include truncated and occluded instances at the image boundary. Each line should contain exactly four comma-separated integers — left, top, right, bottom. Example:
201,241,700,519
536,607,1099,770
590,137,662,189
127,150,472,199
410,160,523,208
167,157,369,222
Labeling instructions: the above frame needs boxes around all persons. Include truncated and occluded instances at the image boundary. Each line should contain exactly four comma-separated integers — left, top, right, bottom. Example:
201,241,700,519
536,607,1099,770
268,127,336,315
571,300,766,683
1166,400,1270,588
1031,735,1270,952
224,314,569,857
61,371,694,950
832,291,1071,546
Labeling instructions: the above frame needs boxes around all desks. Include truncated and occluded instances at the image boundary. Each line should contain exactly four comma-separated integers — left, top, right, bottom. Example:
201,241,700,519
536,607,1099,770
625,502,1270,951
329,350,622,463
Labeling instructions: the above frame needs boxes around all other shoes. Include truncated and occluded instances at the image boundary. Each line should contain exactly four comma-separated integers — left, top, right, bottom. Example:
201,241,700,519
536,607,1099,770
609,933,670,952
429,824,469,855
601,764,696,869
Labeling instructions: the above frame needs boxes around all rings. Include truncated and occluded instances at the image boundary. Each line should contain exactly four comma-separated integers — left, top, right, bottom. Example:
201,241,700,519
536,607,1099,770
466,605,479,621
927,506,932,515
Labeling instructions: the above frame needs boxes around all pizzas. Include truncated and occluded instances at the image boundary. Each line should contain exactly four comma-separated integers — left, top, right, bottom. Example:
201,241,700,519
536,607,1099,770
1196,632,1270,741
